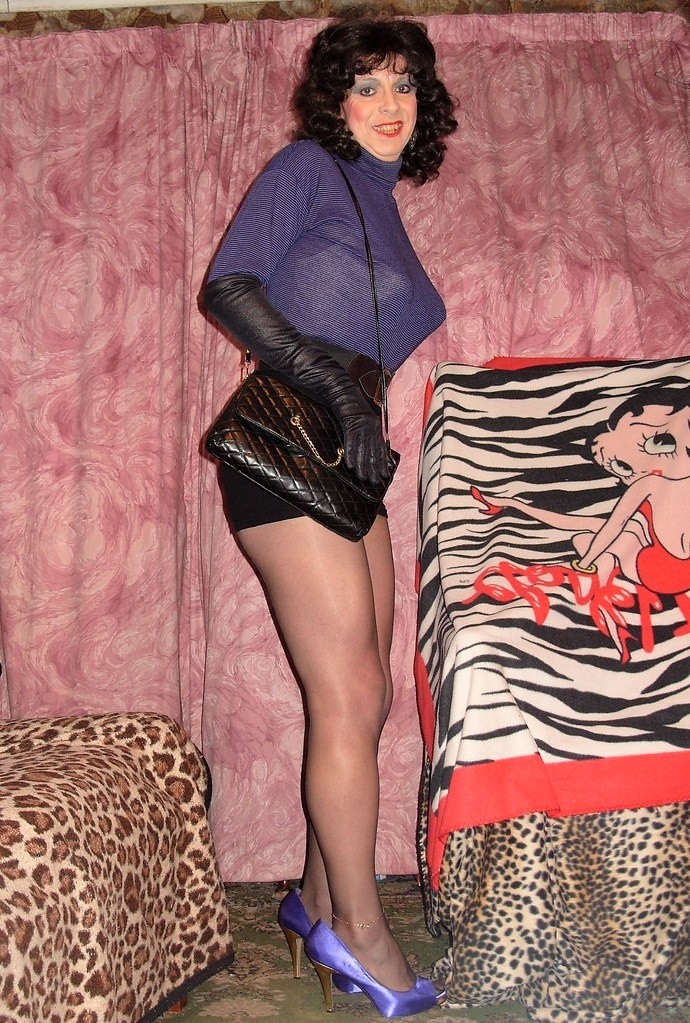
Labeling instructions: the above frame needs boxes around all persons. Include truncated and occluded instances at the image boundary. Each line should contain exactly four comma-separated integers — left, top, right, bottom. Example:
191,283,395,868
196,5,458,1018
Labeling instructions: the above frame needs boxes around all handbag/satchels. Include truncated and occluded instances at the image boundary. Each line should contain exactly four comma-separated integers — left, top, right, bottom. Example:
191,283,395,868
201,367,400,543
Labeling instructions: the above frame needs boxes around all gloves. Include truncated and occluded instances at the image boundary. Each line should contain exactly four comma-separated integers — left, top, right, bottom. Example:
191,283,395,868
199,271,389,486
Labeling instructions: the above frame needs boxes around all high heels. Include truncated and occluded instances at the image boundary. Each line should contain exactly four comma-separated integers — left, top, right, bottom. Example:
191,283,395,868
304,918,445,1018
278,888,363,993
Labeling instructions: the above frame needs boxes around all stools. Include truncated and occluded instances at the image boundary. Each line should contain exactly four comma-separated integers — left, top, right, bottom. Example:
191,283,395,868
0,712,234,1023
